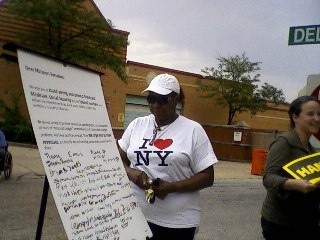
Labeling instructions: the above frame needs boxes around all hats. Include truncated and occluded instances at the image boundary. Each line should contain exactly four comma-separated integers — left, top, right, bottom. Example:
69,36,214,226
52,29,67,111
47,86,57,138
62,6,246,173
141,73,180,95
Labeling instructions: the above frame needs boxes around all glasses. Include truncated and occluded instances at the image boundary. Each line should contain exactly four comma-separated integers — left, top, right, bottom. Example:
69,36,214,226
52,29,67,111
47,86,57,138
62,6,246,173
147,178,159,204
149,128,157,145
147,95,170,105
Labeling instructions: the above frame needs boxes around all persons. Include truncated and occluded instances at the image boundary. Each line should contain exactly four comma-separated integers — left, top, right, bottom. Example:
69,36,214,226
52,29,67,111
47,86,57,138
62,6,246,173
261,95,320,240
114,74,219,240
